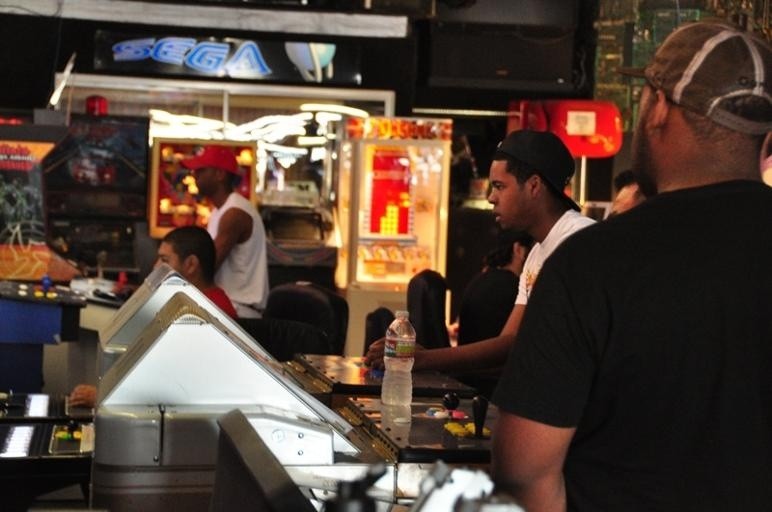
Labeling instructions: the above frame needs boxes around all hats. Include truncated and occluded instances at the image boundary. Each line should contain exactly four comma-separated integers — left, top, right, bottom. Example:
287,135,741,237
616,19,771,137
181,146,242,176
497,129,581,211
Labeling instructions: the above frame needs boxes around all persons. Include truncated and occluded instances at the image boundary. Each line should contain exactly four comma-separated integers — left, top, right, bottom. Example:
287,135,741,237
365,128,652,391
485,19,772,512
179,144,270,336
67,224,240,409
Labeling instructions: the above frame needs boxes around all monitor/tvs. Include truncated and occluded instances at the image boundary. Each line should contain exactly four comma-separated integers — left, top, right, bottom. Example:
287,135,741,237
265,209,327,246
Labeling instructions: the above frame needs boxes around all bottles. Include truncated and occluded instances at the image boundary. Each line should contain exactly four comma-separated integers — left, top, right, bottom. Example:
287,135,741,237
381,311,417,406
